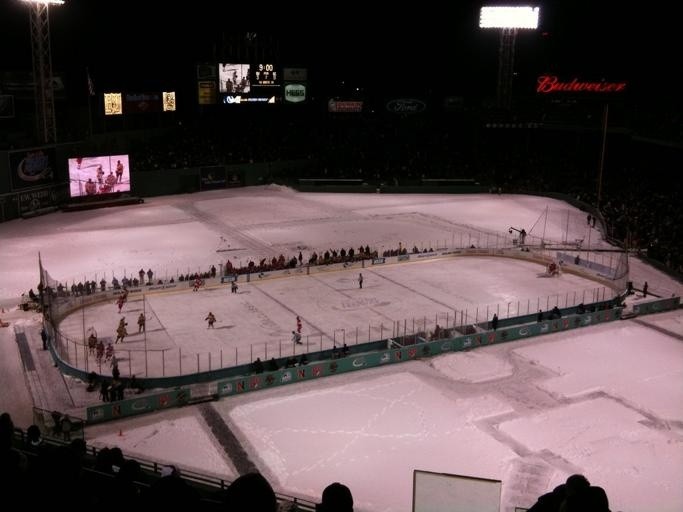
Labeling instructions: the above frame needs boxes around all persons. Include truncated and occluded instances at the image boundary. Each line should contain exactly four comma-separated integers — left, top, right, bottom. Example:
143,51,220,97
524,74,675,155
104,171,116,193
234,84,242,94
575,253,580,264
240,77,247,91
95,164,103,184
643,281,649,297
225,78,232,93
25,244,380,403
114,160,122,184
83,178,95,193
433,292,627,341
132,129,580,198
580,176,683,280
383,243,436,257
232,70,238,84
528,474,610,512
0,412,355,512
